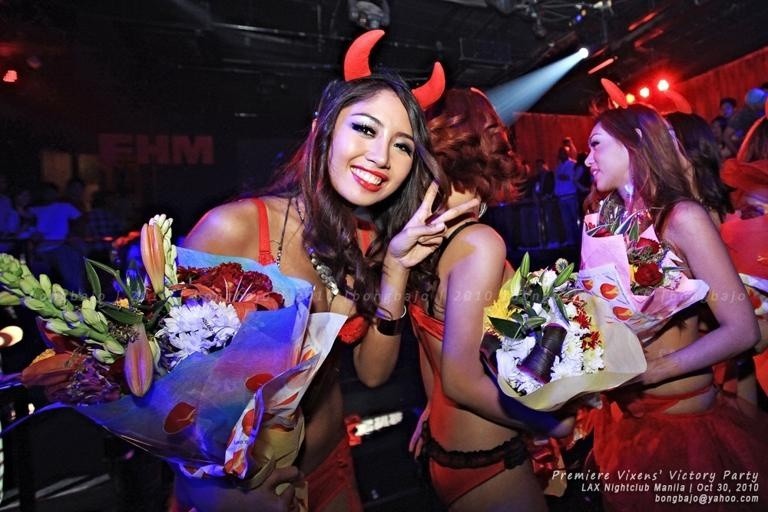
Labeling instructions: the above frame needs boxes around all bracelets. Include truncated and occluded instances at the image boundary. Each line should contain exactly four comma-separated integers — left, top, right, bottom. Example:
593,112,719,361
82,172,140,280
370,305,408,337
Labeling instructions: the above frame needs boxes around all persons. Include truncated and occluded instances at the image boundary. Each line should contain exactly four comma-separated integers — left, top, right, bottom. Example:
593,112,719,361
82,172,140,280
576,102,767,510
406,85,584,512
653,109,766,413
1,76,766,290
166,66,480,512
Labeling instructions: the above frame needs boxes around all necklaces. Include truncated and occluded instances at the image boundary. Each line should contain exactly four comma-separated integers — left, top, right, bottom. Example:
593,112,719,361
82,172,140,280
294,195,342,298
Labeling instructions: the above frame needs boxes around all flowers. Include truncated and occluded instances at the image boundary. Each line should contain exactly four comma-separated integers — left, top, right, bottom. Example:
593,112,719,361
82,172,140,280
0,212,290,508
